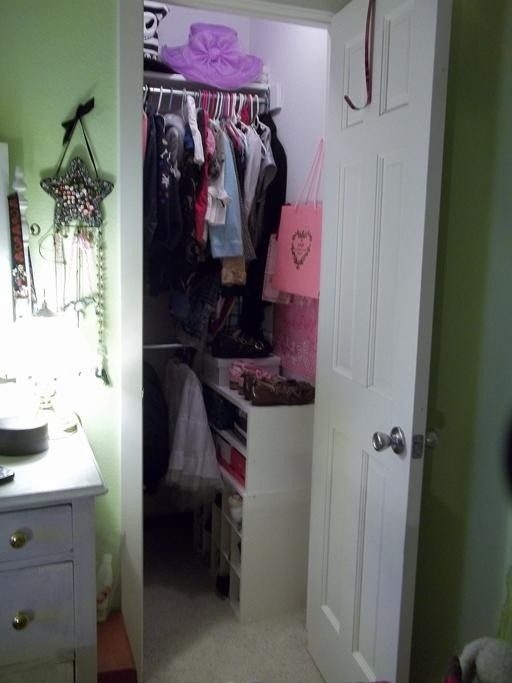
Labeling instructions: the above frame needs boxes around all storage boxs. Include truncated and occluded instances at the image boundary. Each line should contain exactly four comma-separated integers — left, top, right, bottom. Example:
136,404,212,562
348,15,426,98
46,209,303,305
200,351,282,387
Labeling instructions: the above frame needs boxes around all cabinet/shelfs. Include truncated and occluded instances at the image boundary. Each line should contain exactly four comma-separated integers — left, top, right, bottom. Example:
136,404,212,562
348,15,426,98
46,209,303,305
0,411,108,682
198,377,316,625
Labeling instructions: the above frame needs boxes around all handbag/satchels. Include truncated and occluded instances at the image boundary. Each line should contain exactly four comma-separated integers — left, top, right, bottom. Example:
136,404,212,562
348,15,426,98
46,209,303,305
261,205,322,307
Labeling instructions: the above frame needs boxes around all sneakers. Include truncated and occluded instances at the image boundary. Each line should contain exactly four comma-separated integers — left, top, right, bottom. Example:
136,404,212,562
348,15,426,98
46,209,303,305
211,326,273,358
228,360,314,406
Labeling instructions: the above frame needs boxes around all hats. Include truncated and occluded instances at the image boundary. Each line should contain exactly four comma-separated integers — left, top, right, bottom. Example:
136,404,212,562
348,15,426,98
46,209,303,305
161,23,263,91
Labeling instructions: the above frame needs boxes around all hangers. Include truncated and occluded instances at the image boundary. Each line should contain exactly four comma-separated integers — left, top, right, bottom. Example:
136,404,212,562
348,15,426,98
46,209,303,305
144,84,273,159
